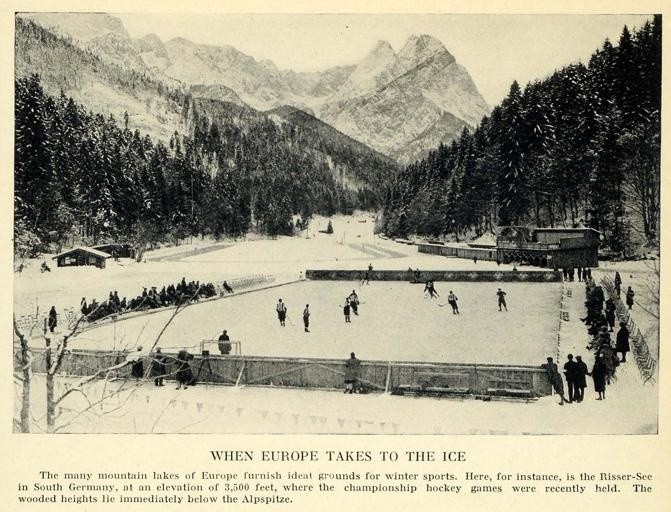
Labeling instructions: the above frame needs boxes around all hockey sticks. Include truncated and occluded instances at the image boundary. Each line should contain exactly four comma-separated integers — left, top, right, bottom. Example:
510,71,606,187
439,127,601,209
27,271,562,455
437,303,449,306
359,302,367,304
286,314,295,326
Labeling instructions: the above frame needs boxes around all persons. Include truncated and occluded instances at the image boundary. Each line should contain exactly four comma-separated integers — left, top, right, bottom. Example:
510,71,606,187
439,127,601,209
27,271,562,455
496,288,507,311
408,267,412,271
223,281,234,293
303,304,310,332
344,352,361,394
218,330,231,354
151,347,166,386
368,264,373,270
503,255,547,268
131,345,144,386
276,298,287,327
424,278,440,299
362,271,369,285
448,291,459,314
344,290,359,322
81,277,217,323
554,264,634,405
496,258,500,265
541,357,568,405
474,256,477,264
48,306,57,332
414,268,420,283
175,349,197,390
